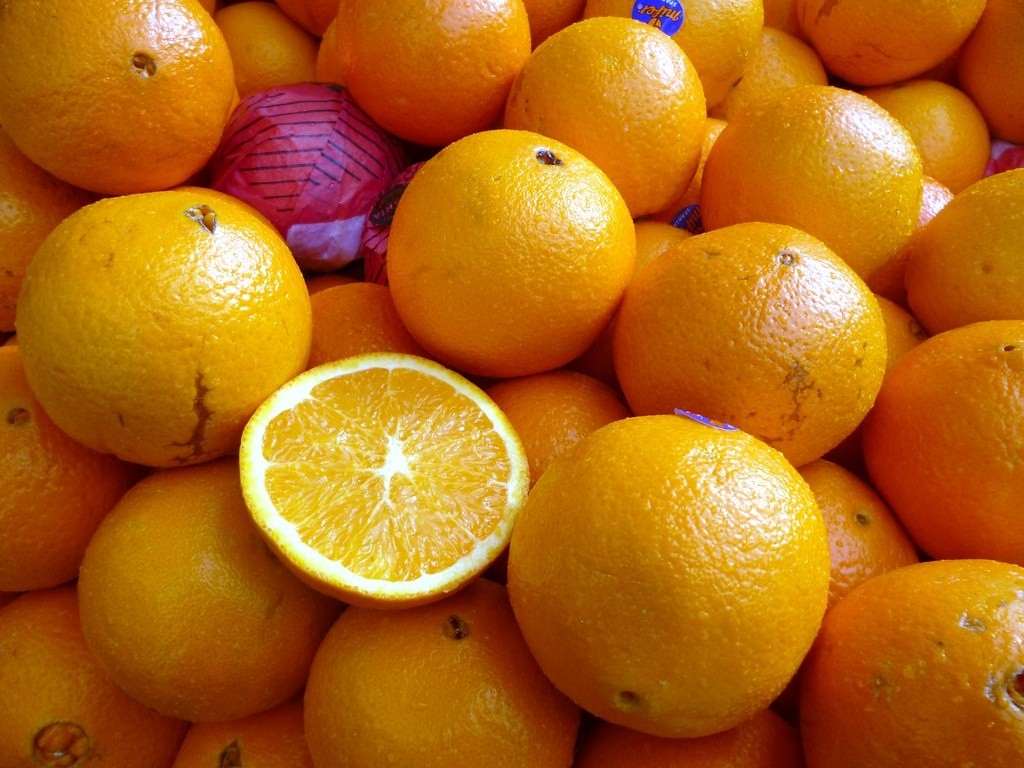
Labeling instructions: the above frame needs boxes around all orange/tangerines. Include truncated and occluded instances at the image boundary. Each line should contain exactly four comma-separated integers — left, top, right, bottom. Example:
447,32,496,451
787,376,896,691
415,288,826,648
0,0,1024,768
